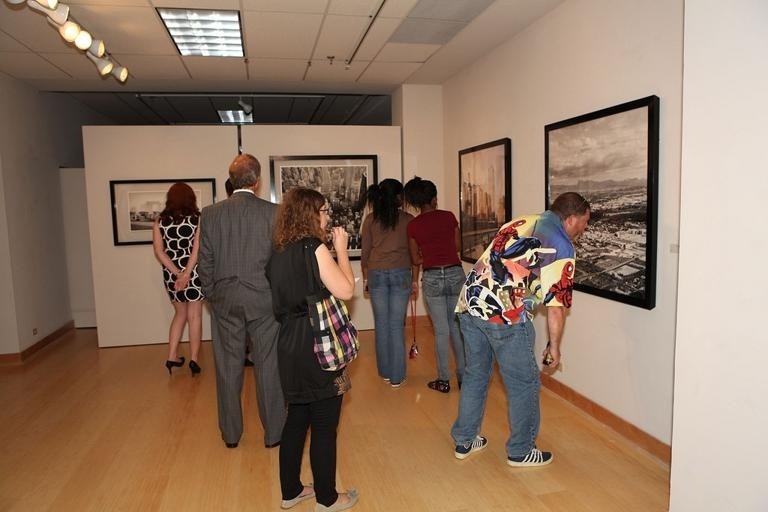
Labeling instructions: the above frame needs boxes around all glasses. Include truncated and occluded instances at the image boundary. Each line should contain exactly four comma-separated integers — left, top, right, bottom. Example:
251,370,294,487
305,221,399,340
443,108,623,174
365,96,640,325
319,208,329,215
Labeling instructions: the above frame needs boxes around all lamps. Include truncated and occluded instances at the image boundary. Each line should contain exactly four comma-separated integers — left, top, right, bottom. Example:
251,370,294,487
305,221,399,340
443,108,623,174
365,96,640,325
25,0,129,83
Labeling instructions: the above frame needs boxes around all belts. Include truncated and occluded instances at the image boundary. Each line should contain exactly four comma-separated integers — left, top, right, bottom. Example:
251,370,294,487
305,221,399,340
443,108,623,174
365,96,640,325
423,264,462,271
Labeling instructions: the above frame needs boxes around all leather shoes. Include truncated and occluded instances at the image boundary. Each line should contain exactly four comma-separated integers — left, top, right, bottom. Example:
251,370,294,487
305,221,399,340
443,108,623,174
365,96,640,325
316,488,359,512
281,482,317,509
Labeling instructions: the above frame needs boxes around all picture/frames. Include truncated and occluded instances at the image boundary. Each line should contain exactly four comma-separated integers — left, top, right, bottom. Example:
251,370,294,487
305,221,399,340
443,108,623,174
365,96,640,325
269,155,378,260
108,177,217,247
457,137,511,264
542,94,660,311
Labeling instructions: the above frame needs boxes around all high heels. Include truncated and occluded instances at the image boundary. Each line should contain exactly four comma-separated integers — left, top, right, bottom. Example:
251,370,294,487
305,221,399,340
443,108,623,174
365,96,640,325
166,357,185,374
190,360,201,377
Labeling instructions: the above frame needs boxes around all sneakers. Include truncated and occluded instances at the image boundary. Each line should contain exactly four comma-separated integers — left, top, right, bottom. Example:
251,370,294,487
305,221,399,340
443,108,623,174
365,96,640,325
391,376,408,387
506,448,554,468
454,435,488,459
428,380,450,392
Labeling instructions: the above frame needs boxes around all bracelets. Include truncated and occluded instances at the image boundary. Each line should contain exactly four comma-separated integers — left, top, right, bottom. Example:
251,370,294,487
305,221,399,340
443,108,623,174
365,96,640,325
175,270,181,276
363,279,368,282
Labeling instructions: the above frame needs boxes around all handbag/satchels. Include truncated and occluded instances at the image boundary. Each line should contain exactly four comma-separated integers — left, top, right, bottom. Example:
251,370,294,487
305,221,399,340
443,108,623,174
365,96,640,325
303,237,360,373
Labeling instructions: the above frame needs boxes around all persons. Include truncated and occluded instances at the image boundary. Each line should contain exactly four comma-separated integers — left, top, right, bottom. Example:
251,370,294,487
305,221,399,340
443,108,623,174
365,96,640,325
198,153,284,448
363,176,418,388
264,187,363,511
224,178,256,369
150,183,206,380
400,177,468,393
449,191,592,469
